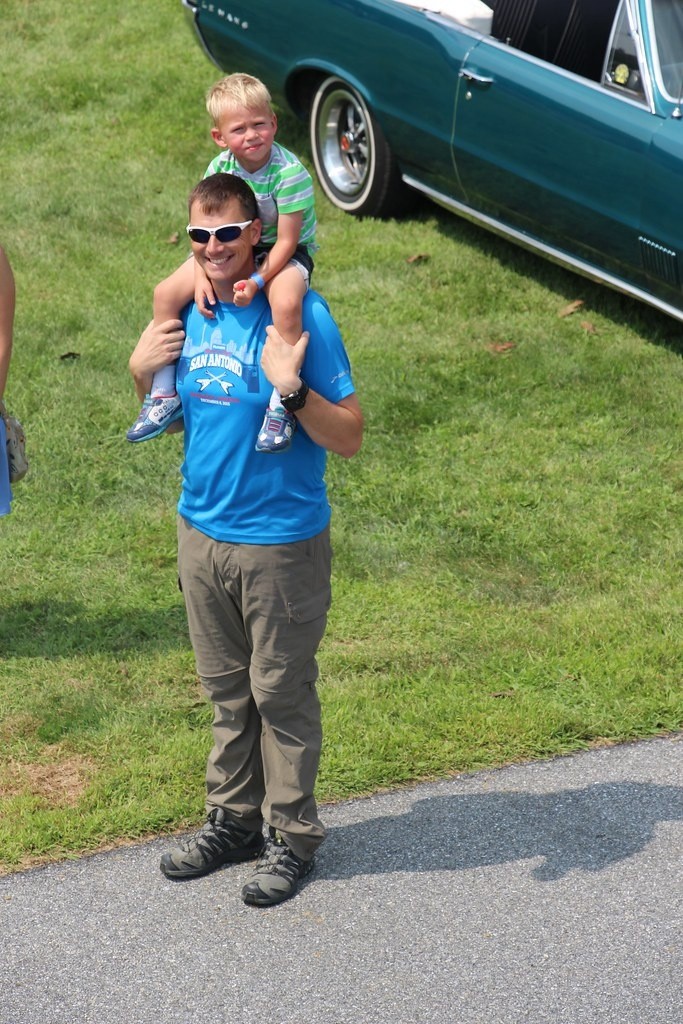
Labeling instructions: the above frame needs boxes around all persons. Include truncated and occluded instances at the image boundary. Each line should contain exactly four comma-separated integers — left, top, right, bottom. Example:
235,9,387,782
123,71,318,454
130,171,366,908
0,249,17,516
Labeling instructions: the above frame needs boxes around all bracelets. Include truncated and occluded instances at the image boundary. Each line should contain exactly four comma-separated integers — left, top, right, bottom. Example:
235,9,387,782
249,272,265,289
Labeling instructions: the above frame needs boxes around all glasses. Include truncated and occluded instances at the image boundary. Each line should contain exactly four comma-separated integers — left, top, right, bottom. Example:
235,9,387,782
186,220,252,243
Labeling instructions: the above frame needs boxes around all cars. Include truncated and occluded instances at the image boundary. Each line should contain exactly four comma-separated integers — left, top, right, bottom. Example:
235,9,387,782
180,0,683,322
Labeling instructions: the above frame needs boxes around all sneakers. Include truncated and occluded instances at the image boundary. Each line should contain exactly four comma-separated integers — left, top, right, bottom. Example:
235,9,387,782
241,825,315,904
255,408,296,454
159,807,264,876
127,391,183,443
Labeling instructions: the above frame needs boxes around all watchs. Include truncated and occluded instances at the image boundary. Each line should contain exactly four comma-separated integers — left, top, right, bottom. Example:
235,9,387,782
279,377,309,413
